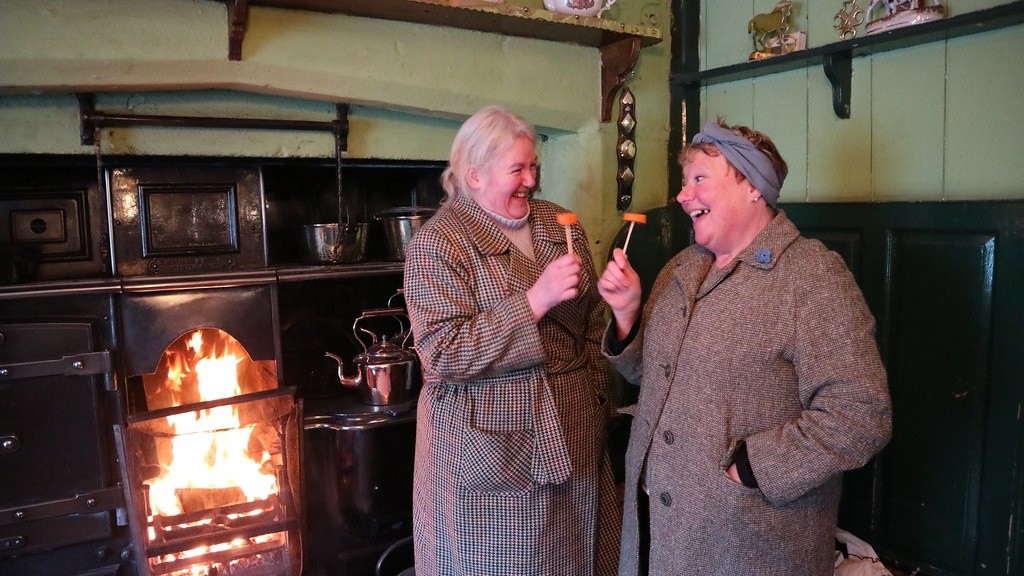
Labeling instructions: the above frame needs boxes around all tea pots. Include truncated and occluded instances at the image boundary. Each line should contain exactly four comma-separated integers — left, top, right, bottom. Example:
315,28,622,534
543,0,616,18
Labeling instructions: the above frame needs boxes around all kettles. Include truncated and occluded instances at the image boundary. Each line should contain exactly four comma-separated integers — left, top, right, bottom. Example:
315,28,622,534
326,310,418,406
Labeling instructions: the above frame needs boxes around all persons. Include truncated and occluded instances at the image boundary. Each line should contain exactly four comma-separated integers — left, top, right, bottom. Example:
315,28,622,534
596,121,893,576
402,105,621,576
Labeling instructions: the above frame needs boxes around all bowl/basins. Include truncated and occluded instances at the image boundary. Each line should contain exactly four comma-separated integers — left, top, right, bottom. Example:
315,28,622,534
375,212,433,261
304,223,367,264
